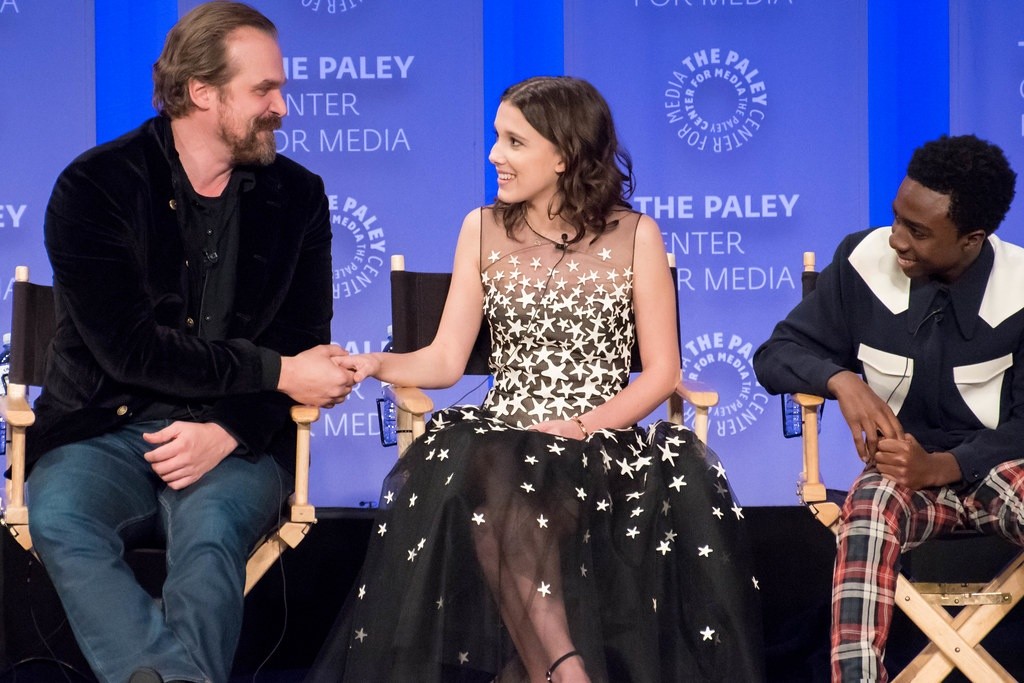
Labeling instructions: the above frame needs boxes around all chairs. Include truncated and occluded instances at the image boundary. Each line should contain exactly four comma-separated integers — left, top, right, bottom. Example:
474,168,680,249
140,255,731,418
0,267,320,599
790,251,1024,683
383,253,721,461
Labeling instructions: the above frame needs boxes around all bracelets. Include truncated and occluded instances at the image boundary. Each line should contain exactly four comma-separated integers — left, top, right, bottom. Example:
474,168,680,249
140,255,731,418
571,417,588,439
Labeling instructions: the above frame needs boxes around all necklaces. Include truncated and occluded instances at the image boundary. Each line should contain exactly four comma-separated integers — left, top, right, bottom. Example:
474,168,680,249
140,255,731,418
527,214,576,246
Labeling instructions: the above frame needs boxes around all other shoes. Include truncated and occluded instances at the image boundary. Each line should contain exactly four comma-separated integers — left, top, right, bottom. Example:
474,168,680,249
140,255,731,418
127,667,164,683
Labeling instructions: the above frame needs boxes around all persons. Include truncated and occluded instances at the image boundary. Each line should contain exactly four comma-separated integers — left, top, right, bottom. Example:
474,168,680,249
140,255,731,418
753,133,1024,683
306,75,760,683
0,1,356,682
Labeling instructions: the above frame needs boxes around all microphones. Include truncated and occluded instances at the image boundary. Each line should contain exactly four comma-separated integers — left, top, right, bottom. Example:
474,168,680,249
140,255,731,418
203,248,210,257
561,233,568,243
938,304,946,313
